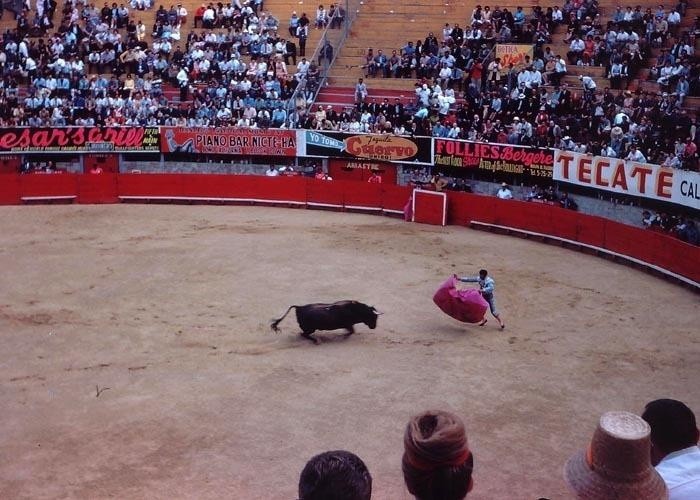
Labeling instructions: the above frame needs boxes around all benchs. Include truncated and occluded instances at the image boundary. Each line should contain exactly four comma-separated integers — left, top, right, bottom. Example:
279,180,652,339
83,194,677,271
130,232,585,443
0,0,699,121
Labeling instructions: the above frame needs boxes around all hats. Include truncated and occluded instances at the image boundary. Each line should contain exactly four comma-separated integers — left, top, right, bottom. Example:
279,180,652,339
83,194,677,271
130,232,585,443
563,410,668,500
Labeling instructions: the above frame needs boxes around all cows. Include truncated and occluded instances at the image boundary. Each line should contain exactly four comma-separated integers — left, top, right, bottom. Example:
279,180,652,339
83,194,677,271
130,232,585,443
270,299,386,345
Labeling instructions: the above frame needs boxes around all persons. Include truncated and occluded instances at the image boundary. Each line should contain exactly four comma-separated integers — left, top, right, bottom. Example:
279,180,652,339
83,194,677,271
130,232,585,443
299,450,372,500
402,448,474,500
454,270,504,329
538,399,700,500
